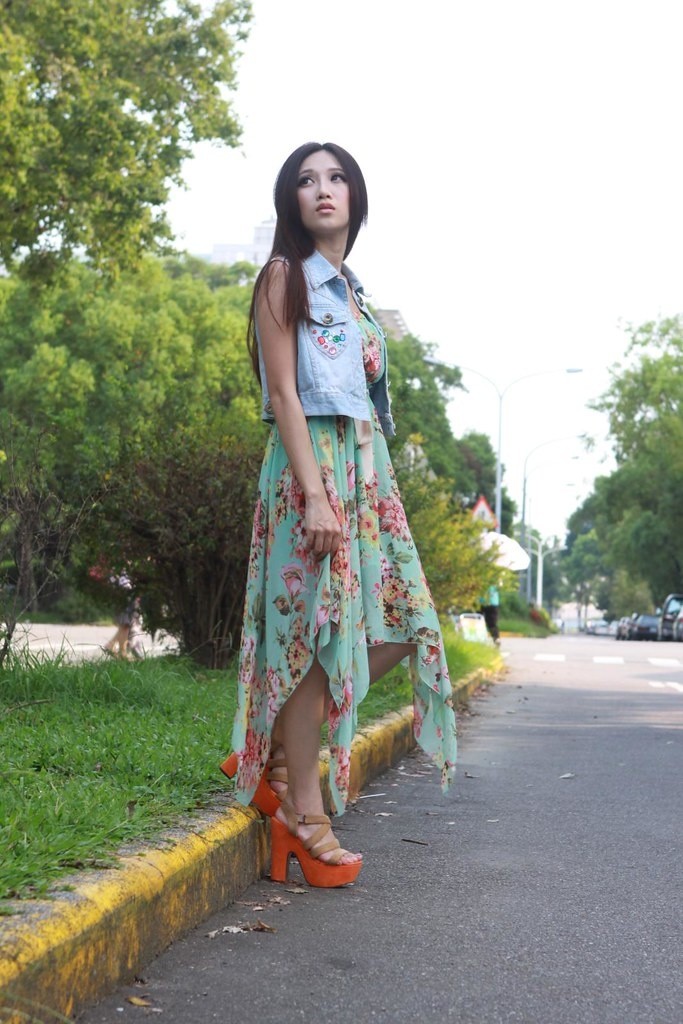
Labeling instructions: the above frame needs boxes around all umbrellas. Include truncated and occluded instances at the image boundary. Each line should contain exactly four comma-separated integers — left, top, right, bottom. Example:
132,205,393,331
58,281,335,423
482,531,531,570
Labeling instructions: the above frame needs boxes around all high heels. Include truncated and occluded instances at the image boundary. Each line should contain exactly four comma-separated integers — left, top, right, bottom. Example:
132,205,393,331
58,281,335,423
221,742,288,818
270,799,360,887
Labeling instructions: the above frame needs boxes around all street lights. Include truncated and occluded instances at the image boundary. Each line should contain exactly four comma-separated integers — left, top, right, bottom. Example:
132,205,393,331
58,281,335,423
494,366,581,537
522,530,569,614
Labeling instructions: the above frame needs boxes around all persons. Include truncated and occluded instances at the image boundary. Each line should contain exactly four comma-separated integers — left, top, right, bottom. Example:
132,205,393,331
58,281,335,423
98,563,146,658
478,570,507,646
529,599,543,626
214,141,460,888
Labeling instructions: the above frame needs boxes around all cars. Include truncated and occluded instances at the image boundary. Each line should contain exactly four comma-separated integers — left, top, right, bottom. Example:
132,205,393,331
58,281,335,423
585,591,682,643
